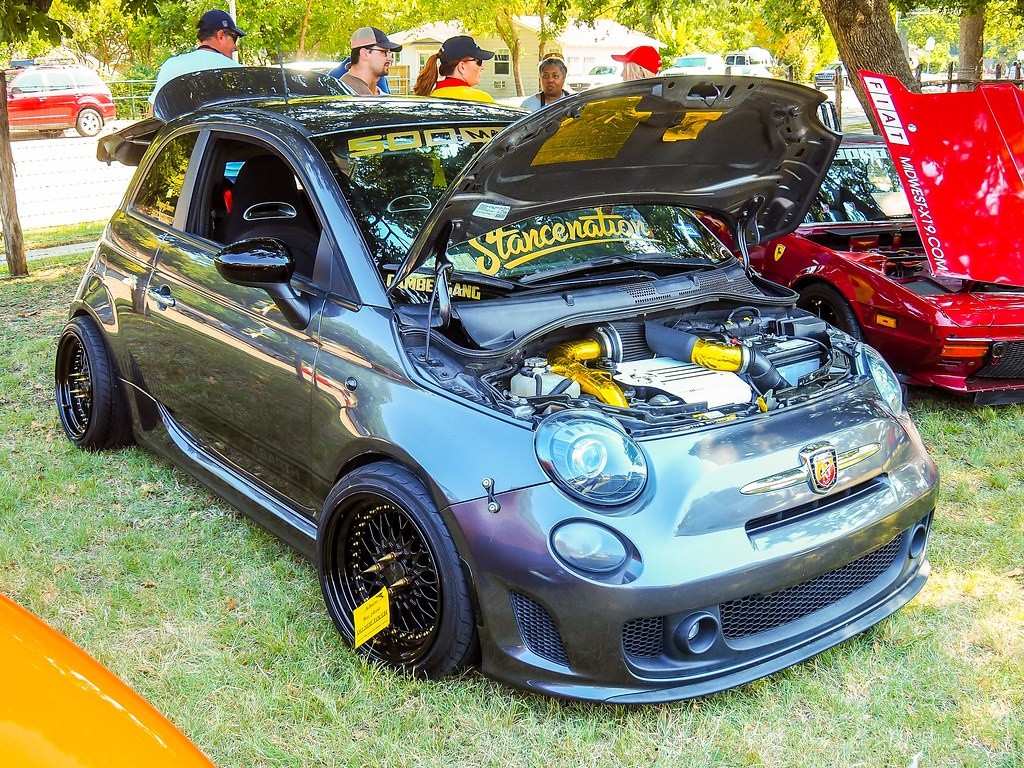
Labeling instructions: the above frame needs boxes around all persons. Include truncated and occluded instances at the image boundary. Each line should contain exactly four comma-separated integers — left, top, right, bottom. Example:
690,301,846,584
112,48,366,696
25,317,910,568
611,46,663,81
148,11,247,118
519,53,576,112
327,27,403,96
414,35,496,103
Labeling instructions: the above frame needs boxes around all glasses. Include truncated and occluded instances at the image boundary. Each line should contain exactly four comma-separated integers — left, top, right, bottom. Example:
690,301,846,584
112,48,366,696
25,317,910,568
460,59,483,66
225,33,237,43
363,47,390,56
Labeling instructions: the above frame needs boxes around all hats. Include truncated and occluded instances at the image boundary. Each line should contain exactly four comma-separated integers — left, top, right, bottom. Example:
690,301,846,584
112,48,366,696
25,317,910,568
439,36,494,64
350,27,402,52
540,53,564,63
611,46,663,75
197,7,247,37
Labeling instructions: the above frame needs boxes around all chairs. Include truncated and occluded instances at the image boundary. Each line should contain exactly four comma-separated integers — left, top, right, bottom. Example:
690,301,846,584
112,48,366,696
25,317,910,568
225,157,319,279
371,151,448,263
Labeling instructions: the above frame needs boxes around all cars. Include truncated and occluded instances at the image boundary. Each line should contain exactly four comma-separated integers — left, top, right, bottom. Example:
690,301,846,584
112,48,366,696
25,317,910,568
54,66,941,707
698,65,1024,410
658,47,776,80
4,57,117,137
565,62,627,93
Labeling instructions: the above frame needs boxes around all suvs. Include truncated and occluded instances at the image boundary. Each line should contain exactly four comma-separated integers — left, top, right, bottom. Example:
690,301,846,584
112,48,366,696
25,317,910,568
814,61,849,91
